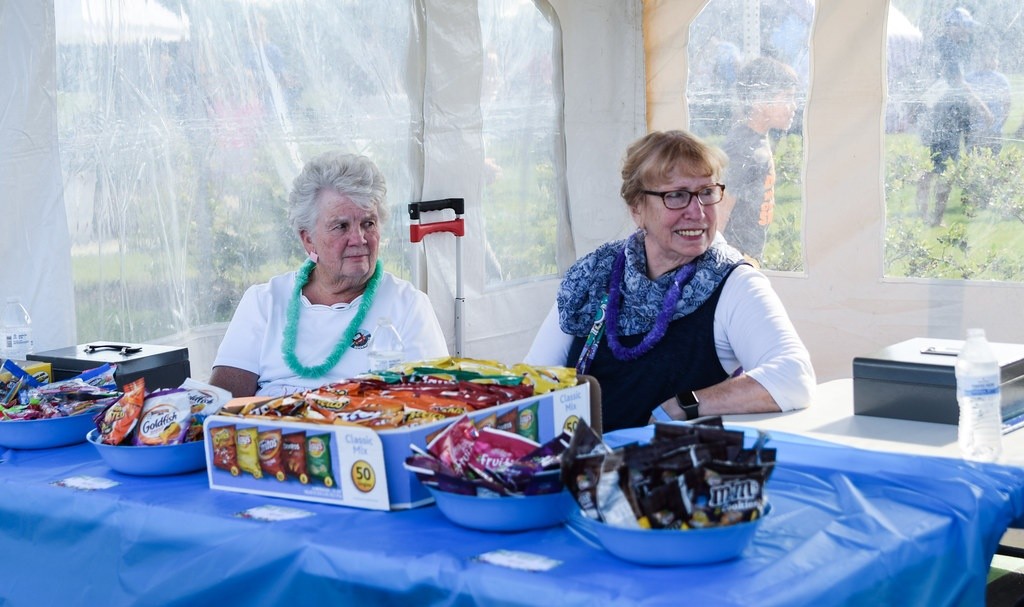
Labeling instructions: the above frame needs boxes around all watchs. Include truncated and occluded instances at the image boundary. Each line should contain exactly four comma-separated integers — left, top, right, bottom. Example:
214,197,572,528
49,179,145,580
675,390,700,421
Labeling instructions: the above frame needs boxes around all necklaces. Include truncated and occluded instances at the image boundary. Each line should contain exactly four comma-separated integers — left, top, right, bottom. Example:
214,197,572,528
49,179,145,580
606,241,696,362
281,257,384,380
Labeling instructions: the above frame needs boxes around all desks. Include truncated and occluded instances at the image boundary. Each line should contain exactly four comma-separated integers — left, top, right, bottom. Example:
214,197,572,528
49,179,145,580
0,371,1024,607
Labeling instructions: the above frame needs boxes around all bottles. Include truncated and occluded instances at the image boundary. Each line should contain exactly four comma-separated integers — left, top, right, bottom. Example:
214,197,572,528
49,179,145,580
954,327,1004,462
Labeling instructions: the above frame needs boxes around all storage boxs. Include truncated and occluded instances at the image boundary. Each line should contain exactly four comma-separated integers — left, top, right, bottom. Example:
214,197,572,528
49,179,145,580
201,373,604,513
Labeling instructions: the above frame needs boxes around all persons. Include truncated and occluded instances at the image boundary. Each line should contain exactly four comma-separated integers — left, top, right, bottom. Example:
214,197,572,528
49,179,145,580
522,131,817,434
207,157,452,398
706,35,797,269
916,6,1012,229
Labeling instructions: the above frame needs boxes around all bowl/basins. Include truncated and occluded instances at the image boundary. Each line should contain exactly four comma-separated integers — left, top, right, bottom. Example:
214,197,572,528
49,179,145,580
572,501,775,564
85,426,209,477
0,411,103,449
422,479,579,532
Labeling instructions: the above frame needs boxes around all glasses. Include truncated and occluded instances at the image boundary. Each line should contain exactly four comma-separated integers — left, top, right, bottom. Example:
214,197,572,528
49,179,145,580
640,183,725,210
